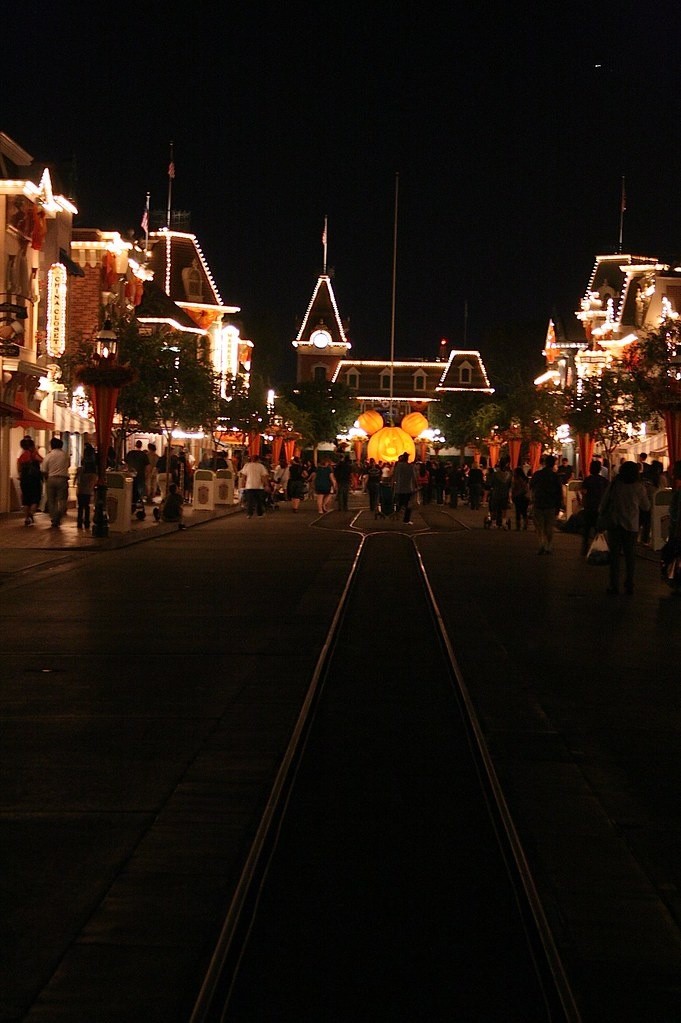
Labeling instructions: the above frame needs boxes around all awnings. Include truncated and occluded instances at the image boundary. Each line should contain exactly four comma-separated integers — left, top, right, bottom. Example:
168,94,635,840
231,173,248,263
54,403,97,436
110,406,162,439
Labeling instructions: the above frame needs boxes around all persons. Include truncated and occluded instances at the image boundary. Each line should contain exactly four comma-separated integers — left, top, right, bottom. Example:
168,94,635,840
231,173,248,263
16,421,680,608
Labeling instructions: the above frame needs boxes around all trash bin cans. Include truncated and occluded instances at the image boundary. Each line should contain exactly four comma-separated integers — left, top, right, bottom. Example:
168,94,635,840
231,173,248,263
214,468,235,505
105,471,134,532
192,470,216,512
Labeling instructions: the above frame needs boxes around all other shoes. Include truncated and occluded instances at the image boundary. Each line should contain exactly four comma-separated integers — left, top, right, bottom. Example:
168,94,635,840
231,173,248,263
606,584,633,594
